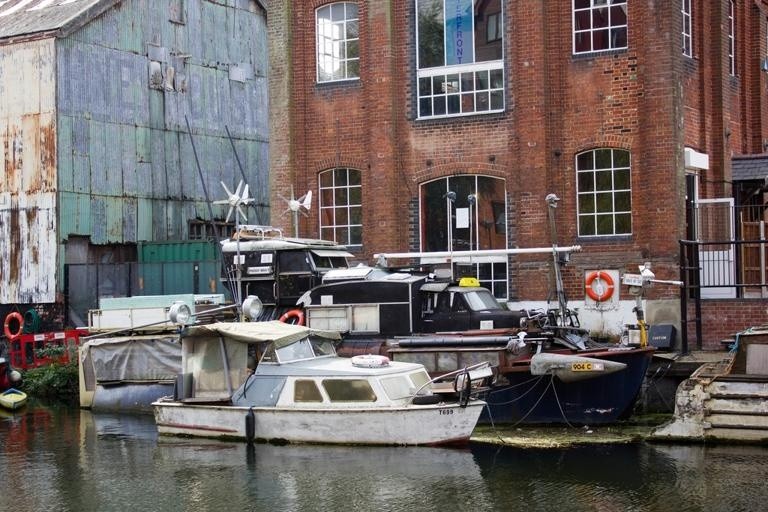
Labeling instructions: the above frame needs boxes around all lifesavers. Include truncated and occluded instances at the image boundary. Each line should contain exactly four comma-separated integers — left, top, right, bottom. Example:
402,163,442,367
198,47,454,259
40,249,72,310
4,312,24,340
279,309,304,326
585,272,614,301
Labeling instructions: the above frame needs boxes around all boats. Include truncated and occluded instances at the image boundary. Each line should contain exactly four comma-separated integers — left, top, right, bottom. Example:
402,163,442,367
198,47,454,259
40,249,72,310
0,387,29,412
0,329,79,393
151,319,497,447
77,114,661,428
